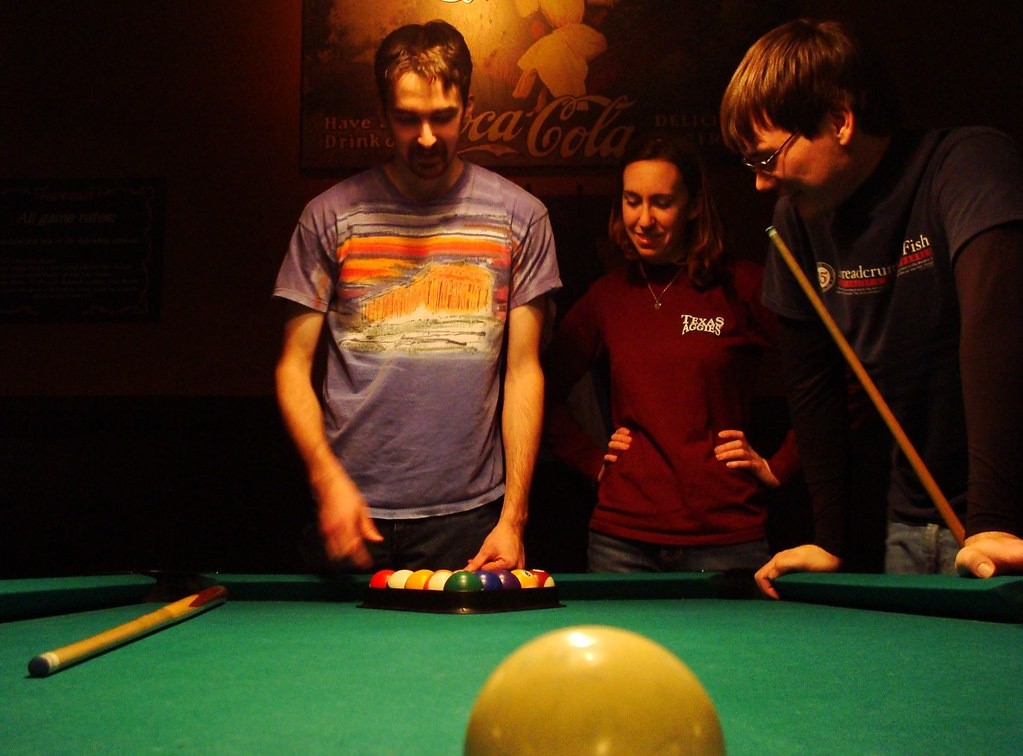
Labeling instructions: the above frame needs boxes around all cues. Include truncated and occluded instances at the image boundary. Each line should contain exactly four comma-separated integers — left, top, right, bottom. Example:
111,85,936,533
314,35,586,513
26,582,229,680
760,222,969,552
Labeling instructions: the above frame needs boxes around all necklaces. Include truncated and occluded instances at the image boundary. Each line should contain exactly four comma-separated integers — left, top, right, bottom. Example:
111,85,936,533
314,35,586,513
640,262,684,310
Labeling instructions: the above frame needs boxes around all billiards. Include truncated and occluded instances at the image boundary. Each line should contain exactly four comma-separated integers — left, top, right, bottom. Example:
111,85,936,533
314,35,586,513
458,621,728,756
367,567,556,593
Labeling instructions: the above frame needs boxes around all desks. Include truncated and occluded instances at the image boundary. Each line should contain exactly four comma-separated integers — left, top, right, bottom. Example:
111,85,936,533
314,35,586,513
0,569,1023,756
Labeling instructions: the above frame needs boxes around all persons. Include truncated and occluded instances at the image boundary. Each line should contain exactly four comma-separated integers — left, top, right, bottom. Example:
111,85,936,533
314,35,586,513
541,133,864,573
720,19,1023,600
271,20,563,573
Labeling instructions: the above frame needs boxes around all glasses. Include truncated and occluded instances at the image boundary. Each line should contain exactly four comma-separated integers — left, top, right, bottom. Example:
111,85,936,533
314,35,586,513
742,128,801,171
